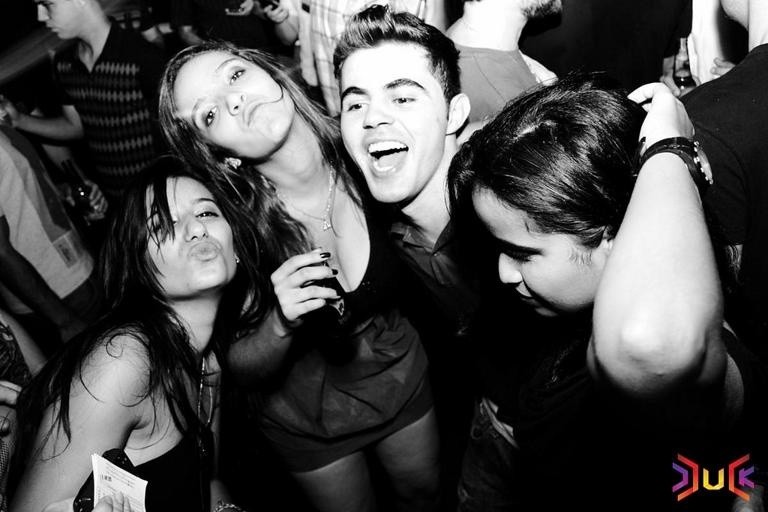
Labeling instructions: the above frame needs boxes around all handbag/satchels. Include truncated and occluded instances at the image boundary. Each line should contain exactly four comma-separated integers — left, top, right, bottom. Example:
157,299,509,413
73,449,155,511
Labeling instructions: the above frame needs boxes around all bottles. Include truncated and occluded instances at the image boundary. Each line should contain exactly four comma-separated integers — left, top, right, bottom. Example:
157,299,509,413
58,158,110,242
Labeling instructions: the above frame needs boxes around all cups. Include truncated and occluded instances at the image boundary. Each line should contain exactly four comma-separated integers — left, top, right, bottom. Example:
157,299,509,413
295,261,356,337
674,54,697,89
